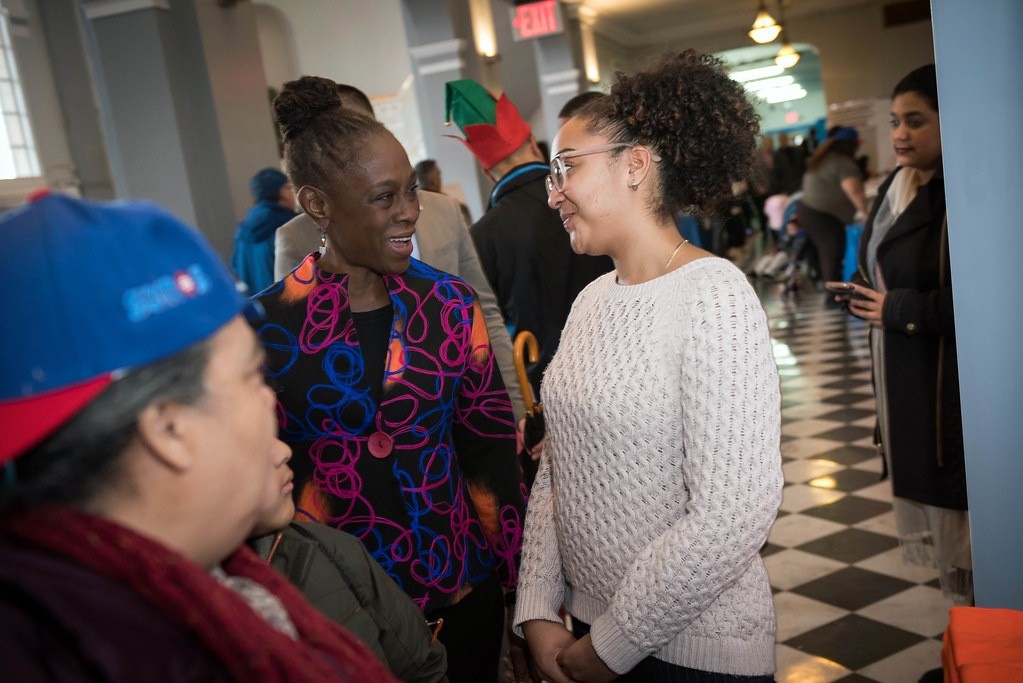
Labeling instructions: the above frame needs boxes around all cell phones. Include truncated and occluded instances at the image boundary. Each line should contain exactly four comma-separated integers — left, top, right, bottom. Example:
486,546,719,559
824,282,868,321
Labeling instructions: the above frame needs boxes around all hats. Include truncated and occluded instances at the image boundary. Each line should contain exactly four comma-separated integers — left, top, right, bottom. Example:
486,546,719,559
1,191,248,468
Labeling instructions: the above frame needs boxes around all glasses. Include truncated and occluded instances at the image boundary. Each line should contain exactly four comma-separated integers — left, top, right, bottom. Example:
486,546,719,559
544,142,663,192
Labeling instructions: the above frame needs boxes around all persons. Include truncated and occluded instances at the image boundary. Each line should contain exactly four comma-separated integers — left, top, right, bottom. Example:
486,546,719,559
228,75,529,682
681,123,823,265
798,123,860,315
227,161,310,313
0,184,403,683
412,156,482,231
440,79,618,397
505,48,783,682
270,77,543,463
833,59,972,597
243,419,451,681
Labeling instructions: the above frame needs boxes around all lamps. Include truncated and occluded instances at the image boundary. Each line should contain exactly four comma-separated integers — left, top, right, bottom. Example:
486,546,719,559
747,1,783,44
774,30,800,69
